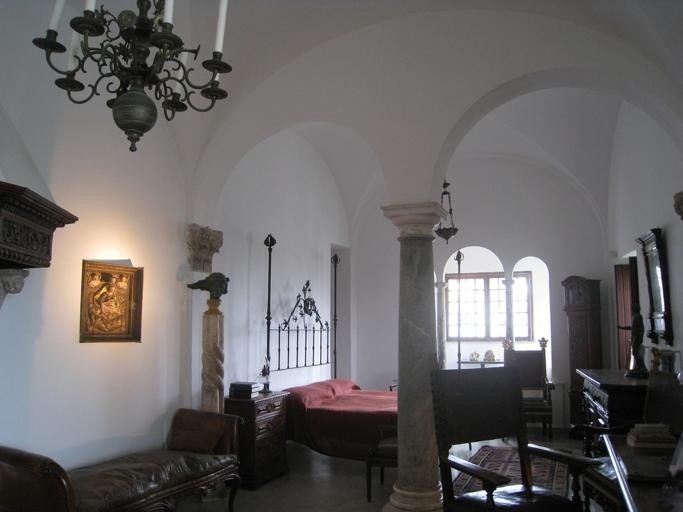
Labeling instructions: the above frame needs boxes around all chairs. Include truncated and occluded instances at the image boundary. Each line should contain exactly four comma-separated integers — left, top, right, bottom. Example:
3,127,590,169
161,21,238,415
502,337,552,439
425,350,601,512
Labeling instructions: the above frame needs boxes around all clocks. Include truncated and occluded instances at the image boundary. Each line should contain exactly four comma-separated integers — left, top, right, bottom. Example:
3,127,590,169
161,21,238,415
560,275,603,432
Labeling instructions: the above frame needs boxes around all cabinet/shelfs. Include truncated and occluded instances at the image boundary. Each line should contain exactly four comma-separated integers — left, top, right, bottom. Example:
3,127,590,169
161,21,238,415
575,367,681,511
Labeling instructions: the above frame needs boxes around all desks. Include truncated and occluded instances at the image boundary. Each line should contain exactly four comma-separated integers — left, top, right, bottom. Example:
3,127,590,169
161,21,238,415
598,433,682,512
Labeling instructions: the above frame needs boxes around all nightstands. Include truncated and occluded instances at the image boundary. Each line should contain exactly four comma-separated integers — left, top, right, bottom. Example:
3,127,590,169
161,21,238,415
223,390,290,491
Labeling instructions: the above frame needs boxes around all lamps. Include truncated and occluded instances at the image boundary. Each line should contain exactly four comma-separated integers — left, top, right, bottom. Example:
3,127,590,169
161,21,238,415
31,0,232,153
432,176,459,244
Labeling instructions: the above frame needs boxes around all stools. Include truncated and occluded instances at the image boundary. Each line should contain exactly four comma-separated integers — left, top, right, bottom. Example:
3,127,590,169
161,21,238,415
364,424,396,503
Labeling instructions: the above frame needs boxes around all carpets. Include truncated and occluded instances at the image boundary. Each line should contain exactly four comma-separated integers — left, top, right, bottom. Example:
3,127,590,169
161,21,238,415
450,446,571,500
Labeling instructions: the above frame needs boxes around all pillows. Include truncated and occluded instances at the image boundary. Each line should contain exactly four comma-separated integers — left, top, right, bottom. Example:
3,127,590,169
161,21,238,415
315,380,361,398
281,384,334,415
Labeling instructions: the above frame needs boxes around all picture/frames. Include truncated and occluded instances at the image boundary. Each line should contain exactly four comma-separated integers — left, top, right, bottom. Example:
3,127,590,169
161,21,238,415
78,260,145,345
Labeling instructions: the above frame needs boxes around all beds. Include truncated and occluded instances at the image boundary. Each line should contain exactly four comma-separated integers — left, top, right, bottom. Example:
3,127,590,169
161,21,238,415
262,232,471,469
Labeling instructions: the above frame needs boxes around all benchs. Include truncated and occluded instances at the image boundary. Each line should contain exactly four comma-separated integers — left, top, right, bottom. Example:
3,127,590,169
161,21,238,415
0,407,245,512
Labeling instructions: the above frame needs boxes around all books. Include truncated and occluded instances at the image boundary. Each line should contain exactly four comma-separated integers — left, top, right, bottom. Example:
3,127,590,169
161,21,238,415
627,423,678,449
229,381,259,399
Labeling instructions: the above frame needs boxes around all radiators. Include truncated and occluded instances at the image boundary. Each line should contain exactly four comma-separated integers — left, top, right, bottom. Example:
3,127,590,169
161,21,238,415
522,384,564,428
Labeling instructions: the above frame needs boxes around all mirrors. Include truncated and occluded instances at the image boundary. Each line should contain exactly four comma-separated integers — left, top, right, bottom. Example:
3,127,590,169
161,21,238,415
633,227,673,348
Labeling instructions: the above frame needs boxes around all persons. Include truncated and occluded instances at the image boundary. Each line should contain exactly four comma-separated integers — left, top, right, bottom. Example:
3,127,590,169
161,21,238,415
615,303,644,370
